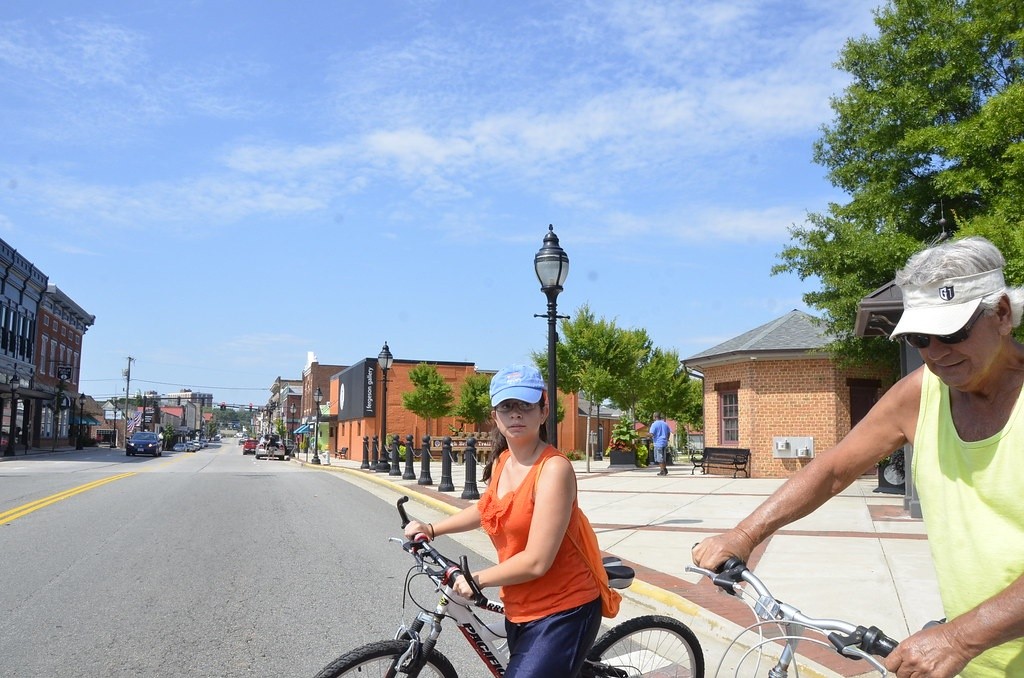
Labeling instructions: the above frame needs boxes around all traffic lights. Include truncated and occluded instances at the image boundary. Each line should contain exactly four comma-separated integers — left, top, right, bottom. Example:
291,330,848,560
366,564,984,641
249,403,253,412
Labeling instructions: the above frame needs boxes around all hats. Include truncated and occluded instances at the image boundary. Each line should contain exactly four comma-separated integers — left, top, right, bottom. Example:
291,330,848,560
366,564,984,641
489,364,546,407
889,267,1005,340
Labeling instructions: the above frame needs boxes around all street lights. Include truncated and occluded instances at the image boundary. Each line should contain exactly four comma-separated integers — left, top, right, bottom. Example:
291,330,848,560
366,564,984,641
375,341,391,473
3,374,20,457
534,223,570,448
76,394,86,450
289,403,296,444
311,385,321,465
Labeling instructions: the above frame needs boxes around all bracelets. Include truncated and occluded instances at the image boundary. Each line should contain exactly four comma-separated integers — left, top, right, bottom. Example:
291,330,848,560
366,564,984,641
428,523,434,541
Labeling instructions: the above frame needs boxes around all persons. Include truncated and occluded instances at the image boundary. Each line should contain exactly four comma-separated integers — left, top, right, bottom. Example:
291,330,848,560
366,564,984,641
401,364,621,678
158,431,165,445
649,411,671,476
266,435,275,461
691,237,1024,678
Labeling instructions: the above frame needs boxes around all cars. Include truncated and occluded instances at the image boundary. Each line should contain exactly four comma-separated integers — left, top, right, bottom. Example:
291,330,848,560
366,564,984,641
255,433,285,461
172,434,223,452
239,439,247,446
242,439,260,456
125,432,164,458
235,433,243,438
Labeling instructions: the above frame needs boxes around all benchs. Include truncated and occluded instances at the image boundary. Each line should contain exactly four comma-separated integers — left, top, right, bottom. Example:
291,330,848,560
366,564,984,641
333,448,348,459
692,447,750,478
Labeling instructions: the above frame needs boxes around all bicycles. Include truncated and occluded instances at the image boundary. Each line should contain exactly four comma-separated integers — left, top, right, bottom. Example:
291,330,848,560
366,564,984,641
685,542,948,677
312,495,705,678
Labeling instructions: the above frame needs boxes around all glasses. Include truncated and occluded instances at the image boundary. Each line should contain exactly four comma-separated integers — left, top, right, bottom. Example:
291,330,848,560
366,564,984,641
902,309,986,348
496,401,538,413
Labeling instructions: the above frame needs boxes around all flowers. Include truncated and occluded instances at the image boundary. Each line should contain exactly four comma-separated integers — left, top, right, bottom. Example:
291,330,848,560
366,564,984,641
604,415,639,455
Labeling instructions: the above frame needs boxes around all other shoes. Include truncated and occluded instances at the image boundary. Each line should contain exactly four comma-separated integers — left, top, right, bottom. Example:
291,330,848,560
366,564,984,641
657,469,668,476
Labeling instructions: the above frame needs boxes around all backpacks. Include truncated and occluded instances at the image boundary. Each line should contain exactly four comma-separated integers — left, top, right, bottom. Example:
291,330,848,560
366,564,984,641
534,453,622,619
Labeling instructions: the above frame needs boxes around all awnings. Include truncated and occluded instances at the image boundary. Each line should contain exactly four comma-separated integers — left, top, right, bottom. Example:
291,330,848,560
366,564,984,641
69,414,101,425
293,425,309,433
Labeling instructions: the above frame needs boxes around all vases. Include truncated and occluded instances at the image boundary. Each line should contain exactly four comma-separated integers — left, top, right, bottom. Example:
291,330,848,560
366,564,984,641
608,450,638,470
